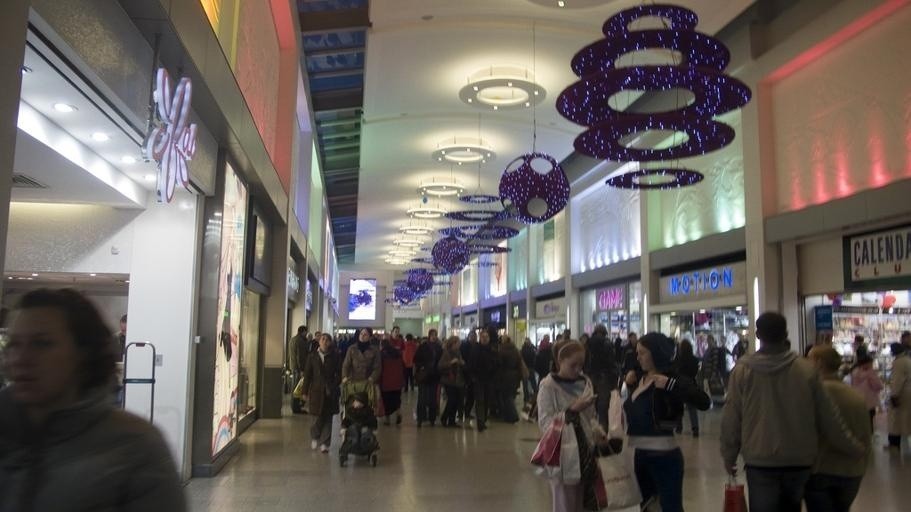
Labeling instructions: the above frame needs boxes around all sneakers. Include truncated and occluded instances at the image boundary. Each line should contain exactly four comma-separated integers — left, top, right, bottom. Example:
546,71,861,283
320,444,329,453
311,438,318,451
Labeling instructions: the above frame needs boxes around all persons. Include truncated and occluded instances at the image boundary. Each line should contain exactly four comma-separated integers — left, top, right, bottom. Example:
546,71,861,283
286,309,911,512
0,285,190,512
112,314,127,410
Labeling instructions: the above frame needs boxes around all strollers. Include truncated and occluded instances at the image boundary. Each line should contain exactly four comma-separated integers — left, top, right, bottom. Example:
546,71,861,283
338,376,381,466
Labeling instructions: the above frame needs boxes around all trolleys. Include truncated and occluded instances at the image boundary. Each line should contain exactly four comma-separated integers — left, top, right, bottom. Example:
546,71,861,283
120,341,157,428
709,375,726,407
523,370,538,413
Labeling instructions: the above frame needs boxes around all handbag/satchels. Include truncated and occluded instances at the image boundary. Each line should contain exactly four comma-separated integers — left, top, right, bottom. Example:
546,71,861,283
293,378,308,400
583,438,643,511
519,357,530,379
723,474,748,511
529,410,598,486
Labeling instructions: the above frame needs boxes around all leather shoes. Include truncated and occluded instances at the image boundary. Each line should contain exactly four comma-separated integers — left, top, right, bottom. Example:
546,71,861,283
884,445,901,451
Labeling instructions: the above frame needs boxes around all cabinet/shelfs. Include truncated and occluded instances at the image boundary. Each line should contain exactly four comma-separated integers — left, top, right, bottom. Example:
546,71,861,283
815,305,910,408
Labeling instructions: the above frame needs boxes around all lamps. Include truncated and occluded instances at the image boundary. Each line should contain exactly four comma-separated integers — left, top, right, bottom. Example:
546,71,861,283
385,179,465,267
459,66,546,112
433,138,496,166
